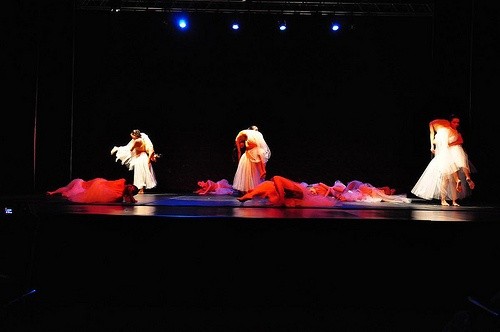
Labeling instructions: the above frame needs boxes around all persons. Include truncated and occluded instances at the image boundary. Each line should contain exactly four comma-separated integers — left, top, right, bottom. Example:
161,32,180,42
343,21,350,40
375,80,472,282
46,178,139,205
193,178,242,195
232,129,272,181
329,180,412,203
237,176,331,204
232,123,266,196
428,113,475,193
111,132,162,165
410,113,468,207
130,129,158,194
